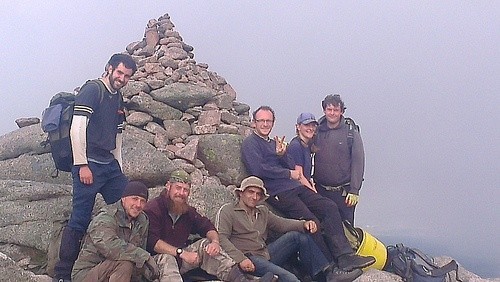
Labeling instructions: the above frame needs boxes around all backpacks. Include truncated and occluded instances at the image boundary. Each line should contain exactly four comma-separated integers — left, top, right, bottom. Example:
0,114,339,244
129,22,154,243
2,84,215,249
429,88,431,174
42,80,123,172
344,222,458,282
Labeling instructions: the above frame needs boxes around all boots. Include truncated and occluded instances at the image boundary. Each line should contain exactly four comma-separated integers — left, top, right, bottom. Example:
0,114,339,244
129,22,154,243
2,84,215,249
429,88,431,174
54,228,82,282
226,264,273,282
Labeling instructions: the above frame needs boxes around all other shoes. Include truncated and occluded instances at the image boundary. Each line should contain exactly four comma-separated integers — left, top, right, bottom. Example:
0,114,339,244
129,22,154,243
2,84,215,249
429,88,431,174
339,255,376,272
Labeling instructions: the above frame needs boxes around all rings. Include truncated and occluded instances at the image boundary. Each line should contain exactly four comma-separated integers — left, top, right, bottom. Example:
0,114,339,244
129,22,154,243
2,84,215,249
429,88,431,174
247,268,249,269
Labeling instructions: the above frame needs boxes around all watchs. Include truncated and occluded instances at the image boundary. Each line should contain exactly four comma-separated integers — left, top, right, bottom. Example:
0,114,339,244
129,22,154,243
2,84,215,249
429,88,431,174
176,248,183,258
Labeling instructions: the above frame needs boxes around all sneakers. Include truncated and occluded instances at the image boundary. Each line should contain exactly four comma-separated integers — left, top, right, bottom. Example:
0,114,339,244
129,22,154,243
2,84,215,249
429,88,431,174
326,266,362,282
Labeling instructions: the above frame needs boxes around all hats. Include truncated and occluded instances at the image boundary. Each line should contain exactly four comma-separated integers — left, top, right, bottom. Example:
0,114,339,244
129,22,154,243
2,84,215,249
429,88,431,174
233,176,270,203
170,171,191,188
122,181,149,201
297,112,319,127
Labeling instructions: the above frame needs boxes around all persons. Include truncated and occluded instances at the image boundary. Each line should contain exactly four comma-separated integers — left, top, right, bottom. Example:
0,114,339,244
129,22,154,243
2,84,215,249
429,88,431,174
54,53,138,282
216,177,363,282
313,94,364,225
142,170,277,282
240,105,376,272
284,112,318,193
71,181,184,282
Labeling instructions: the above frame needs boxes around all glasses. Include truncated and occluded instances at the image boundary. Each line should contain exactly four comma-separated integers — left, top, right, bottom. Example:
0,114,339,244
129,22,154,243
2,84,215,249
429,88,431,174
256,119,273,124
